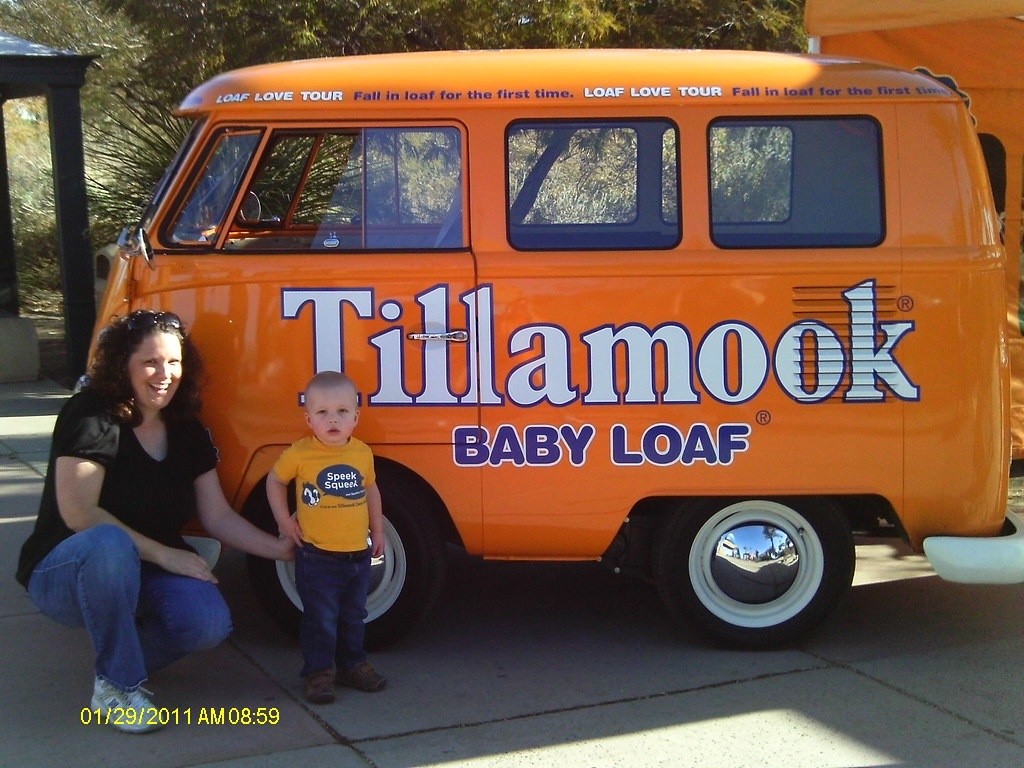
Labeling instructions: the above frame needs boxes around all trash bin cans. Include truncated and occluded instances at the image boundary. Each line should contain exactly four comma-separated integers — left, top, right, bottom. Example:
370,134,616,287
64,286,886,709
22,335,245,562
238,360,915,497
94,244,120,315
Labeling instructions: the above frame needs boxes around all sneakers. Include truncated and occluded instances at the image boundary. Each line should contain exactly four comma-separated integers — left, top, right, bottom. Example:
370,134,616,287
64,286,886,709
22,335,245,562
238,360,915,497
304,669,336,703
341,661,385,691
90,671,164,733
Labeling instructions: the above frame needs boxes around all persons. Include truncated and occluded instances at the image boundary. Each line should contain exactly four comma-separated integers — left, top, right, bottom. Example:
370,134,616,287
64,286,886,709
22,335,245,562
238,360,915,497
16,310,295,731
266,371,388,703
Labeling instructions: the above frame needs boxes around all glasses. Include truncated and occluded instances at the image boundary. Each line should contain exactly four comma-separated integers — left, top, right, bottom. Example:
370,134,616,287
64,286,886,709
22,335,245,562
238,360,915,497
125,311,180,336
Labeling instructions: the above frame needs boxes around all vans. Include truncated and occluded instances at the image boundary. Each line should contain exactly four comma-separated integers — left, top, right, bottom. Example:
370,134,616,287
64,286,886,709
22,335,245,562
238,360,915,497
74,48,1016,656
